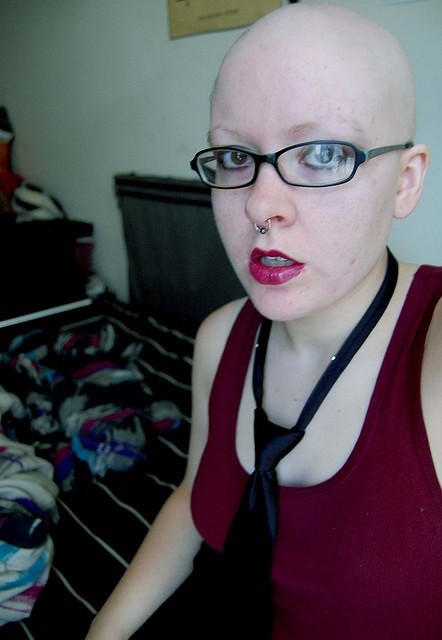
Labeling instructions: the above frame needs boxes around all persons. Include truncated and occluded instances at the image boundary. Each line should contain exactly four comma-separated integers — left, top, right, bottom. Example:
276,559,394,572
84,3,442,640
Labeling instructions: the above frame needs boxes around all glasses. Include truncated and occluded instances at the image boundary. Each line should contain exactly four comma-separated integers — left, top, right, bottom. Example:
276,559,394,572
190,140,413,189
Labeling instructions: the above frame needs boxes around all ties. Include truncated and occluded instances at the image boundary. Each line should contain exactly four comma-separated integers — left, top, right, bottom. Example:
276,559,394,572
217,246,398,639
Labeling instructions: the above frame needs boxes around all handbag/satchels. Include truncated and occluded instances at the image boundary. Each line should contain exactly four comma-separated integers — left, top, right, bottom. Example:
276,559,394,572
1,184,93,321
